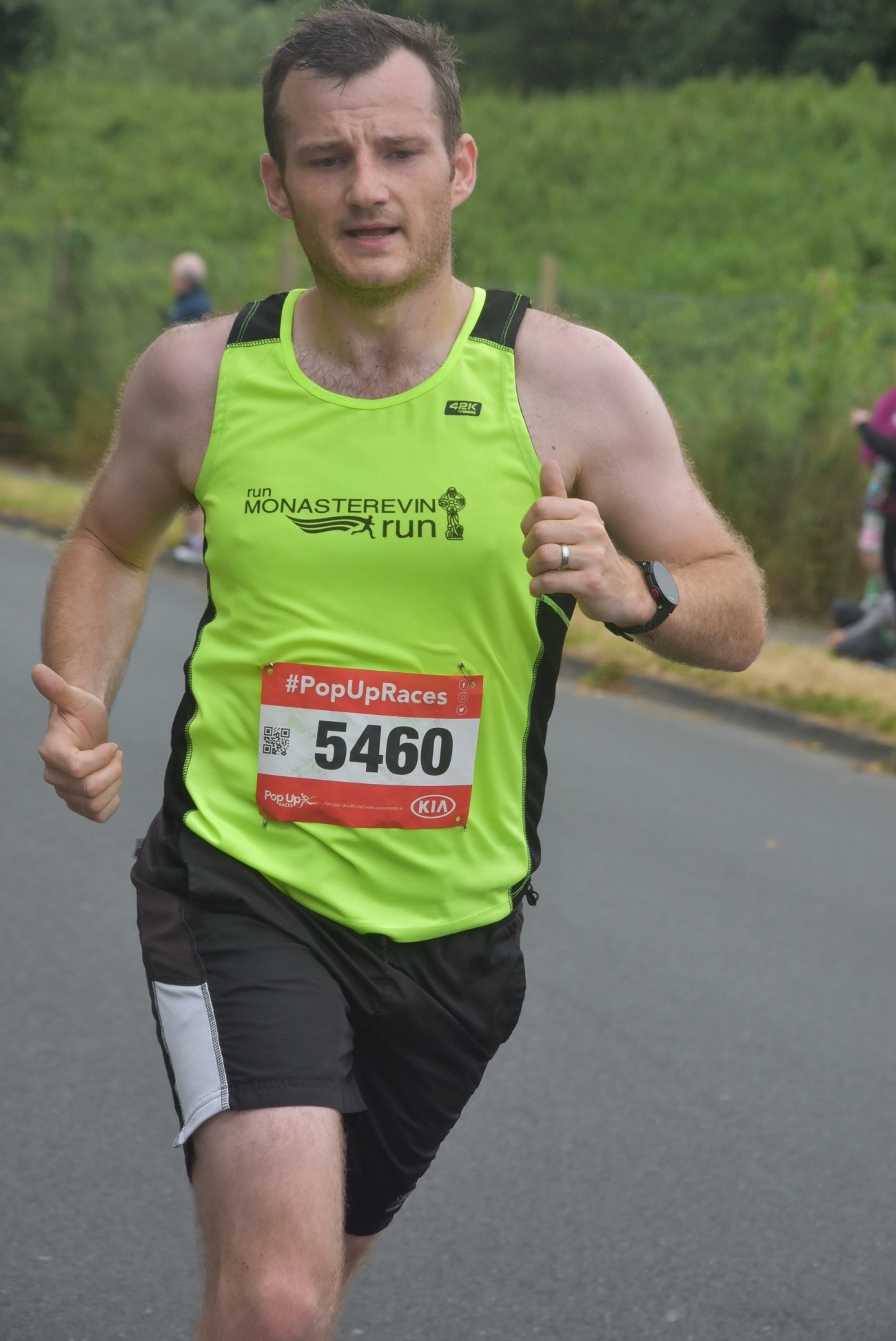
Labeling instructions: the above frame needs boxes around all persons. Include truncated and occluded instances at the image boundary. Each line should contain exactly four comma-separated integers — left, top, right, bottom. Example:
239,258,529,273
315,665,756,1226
166,252,211,564
850,389,896,601
28,7,768,1341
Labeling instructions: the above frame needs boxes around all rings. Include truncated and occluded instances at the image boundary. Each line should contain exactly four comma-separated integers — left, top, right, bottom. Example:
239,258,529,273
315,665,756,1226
558,543,569,571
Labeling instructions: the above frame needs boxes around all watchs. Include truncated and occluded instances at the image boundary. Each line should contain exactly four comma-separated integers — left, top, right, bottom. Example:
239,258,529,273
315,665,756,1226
603,560,680,642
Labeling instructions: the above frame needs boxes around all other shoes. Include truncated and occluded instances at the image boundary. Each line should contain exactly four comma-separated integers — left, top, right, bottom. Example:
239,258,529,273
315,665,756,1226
172,535,204,565
